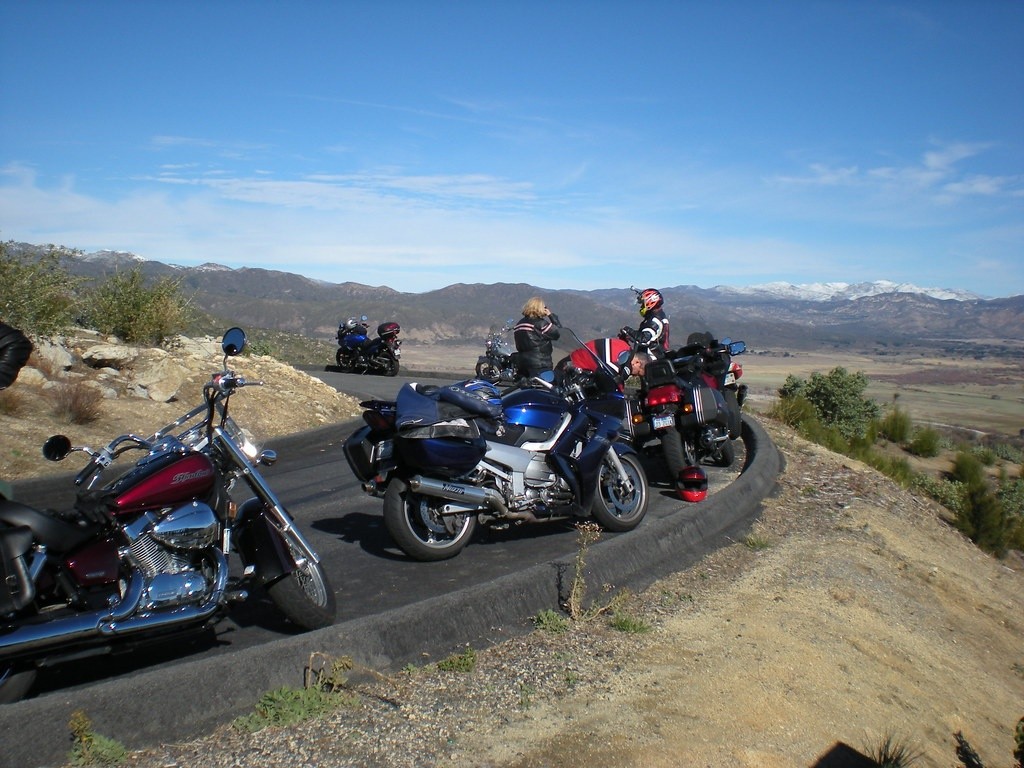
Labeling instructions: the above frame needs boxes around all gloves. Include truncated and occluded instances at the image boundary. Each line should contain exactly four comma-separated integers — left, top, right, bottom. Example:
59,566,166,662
75,489,121,524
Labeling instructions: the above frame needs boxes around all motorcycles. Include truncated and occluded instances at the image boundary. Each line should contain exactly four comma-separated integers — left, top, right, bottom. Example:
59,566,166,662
343,326,649,561
0,327,339,704
327,315,402,377
475,319,527,384
632,330,748,483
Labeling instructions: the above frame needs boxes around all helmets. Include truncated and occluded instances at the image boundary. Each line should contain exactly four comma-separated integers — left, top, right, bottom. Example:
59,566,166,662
677,467,707,502
465,378,502,407
637,289,664,318
347,318,357,329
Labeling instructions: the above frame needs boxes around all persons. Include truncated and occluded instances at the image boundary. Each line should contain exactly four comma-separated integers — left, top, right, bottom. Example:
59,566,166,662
553,338,653,398
514,297,560,390
620,289,670,393
